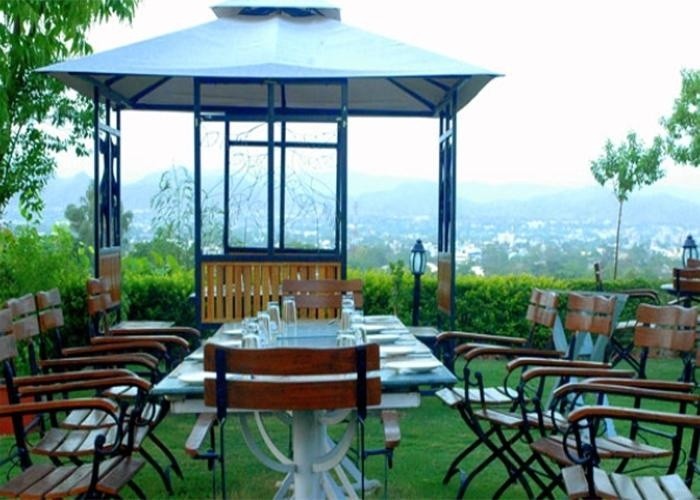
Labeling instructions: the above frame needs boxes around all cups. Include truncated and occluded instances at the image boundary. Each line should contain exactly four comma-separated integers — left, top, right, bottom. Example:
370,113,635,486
334,294,365,347
241,294,297,349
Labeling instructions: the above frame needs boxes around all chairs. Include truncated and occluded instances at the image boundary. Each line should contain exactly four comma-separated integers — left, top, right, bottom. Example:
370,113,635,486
280,279,365,319
182,342,403,500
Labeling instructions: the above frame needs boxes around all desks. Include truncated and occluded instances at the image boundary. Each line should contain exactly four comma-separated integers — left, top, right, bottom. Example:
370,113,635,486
184,280,285,299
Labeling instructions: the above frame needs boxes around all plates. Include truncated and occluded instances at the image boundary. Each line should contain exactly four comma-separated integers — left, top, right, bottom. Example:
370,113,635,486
353,324,444,373
176,327,246,386
